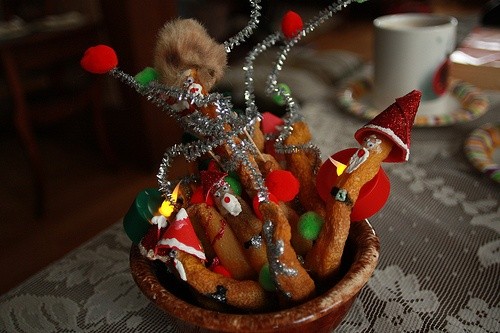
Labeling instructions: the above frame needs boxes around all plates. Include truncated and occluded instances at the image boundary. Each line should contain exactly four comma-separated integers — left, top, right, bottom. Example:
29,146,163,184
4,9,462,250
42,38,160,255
334,66,492,129
461,106,500,189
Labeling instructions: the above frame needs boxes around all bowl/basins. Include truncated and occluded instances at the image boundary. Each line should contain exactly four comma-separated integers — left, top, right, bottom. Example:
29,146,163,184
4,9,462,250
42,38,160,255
128,201,380,333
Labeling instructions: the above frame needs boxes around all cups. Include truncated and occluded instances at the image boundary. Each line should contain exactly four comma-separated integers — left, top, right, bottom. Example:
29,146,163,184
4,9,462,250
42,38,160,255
372,13,458,110
